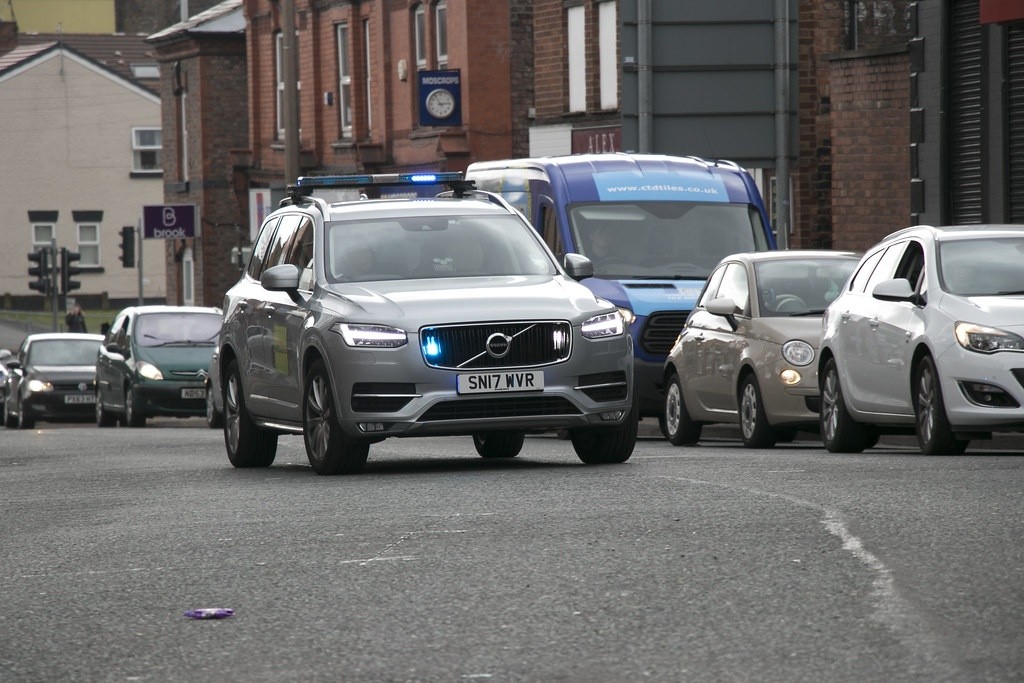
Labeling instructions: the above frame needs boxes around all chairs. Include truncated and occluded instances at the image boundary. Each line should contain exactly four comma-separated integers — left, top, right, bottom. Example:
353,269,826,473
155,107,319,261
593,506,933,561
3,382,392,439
413,232,485,277
300,234,377,282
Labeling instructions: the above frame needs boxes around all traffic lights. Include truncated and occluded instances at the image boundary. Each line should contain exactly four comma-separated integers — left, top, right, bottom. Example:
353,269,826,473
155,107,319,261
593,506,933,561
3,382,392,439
26,246,53,297
61,246,83,294
117,225,133,268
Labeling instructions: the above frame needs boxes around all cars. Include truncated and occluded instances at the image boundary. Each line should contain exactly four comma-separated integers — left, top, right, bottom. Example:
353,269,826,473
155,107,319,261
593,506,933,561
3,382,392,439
4,333,108,429
90,305,224,428
0,348,15,409
658,250,866,449
816,226,1024,454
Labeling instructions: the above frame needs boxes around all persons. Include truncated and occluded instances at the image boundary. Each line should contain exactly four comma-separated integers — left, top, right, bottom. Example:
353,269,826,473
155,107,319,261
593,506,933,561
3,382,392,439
582,222,630,268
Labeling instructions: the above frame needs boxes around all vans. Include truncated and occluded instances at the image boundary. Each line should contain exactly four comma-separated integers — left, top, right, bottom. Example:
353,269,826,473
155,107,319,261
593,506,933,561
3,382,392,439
460,153,780,440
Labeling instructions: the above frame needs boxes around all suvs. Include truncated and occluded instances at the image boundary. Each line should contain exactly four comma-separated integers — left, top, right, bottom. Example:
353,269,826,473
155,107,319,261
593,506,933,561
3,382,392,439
217,172,641,476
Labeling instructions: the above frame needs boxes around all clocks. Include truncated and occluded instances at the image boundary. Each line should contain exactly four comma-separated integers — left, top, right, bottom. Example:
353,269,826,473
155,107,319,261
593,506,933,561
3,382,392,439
425,89,455,119
